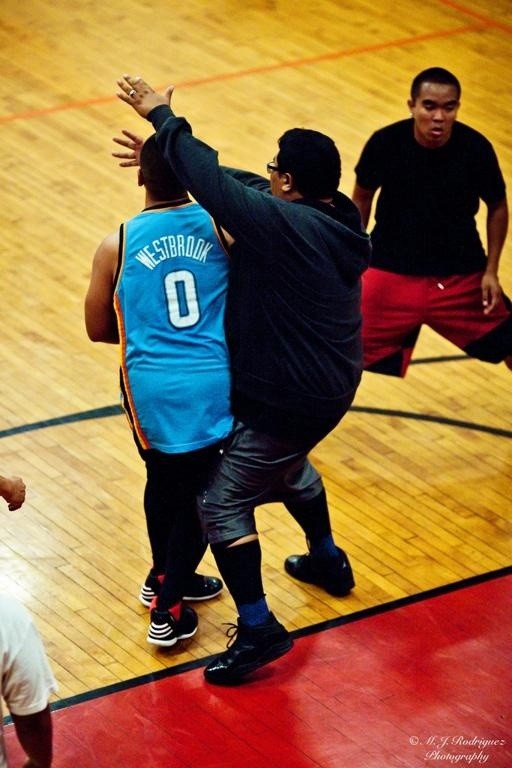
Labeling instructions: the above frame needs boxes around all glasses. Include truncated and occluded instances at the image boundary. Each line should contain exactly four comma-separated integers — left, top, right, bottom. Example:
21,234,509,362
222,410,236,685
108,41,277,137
267,162,280,173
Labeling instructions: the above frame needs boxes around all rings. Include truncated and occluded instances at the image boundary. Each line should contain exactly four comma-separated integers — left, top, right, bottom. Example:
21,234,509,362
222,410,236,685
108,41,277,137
127,89,138,97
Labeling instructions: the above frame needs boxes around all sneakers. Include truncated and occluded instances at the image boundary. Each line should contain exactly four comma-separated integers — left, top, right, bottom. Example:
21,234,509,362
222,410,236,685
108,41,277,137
204,612,293,684
285,546,355,596
141,570,223,646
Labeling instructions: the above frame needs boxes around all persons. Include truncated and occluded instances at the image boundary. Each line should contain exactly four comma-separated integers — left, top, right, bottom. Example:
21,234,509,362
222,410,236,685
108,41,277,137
82,130,237,651
350,65,512,381
1,584,63,767
0,461,28,514
107,72,370,687
